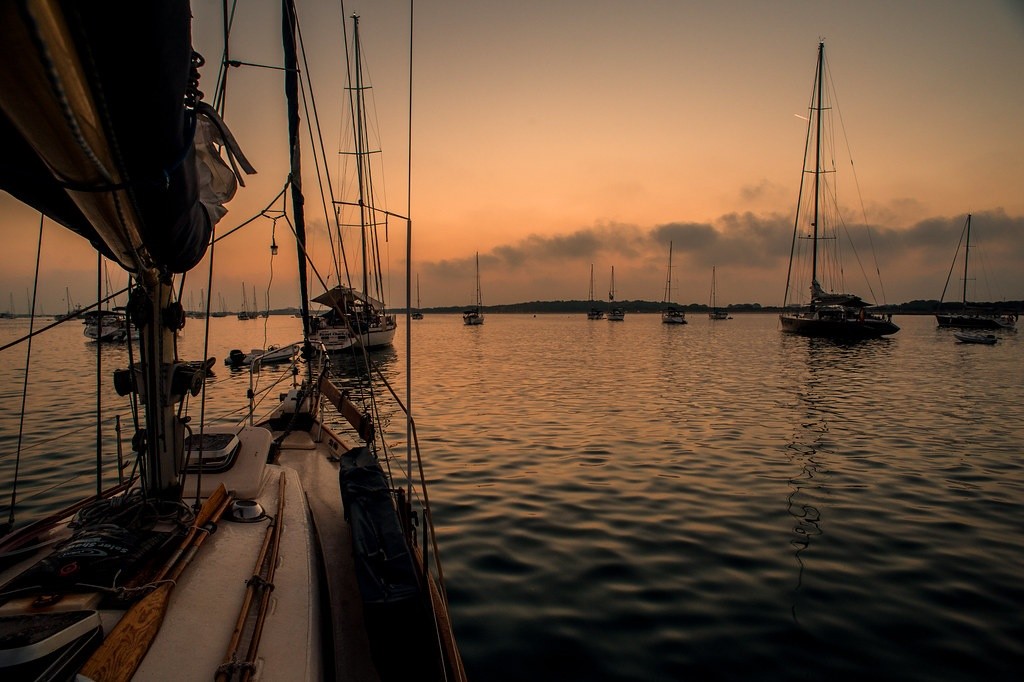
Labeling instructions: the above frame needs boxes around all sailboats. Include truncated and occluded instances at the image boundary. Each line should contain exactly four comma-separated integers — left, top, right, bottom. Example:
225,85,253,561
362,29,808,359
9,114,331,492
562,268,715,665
462,251,488,325
601,263,628,324
9,260,273,342
773,33,902,337
657,236,688,324
705,266,729,319
587,262,605,321
935,211,1021,328
412,273,426,320
313,13,400,350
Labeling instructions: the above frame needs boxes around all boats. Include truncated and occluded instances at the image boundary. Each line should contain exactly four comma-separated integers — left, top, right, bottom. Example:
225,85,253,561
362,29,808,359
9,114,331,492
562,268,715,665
952,330,1000,345
225,343,298,371
